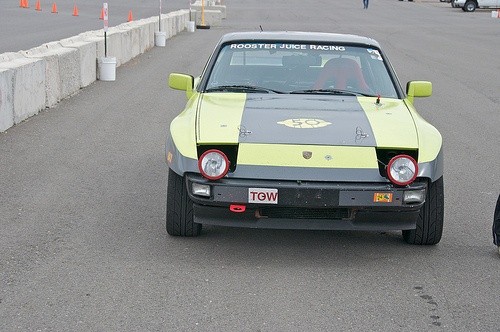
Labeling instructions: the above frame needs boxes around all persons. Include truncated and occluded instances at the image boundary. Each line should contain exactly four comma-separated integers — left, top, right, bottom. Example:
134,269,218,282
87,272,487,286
362,0,369,9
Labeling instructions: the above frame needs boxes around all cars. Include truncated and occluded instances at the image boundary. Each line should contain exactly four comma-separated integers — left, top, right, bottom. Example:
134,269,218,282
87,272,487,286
165,31,446,245
441,0,500,13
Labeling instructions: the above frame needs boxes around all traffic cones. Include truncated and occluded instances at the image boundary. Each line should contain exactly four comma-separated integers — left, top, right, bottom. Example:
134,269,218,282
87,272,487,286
34,0,42,11
98,4,104,20
127,8,133,22
50,1,58,13
19,0,30,8
72,3,79,16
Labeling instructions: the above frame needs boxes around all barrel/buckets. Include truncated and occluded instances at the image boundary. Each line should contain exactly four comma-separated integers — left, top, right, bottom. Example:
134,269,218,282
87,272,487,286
97,57,116,81
491,11,498,18
155,32,165,46
187,21,195,32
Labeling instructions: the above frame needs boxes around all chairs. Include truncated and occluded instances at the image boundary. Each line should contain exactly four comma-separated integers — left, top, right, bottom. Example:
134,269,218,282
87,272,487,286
316,57,371,92
